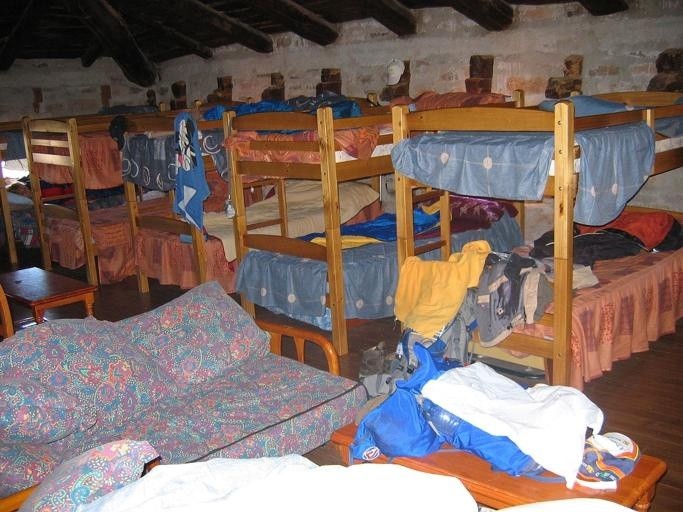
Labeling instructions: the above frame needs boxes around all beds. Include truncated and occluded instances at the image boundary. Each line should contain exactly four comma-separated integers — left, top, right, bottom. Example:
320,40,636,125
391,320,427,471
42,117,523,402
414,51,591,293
0,92,683,385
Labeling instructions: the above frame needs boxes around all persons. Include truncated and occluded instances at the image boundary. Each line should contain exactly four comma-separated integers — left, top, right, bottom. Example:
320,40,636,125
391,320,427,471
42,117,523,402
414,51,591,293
6,179,138,207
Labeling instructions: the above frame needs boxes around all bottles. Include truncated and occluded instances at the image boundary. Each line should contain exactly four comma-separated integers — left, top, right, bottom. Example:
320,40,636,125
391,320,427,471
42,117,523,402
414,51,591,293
420,398,461,439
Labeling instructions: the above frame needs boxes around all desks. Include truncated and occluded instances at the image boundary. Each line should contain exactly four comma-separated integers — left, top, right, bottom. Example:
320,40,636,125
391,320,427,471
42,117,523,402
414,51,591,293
331,393,667,512
0,266,98,326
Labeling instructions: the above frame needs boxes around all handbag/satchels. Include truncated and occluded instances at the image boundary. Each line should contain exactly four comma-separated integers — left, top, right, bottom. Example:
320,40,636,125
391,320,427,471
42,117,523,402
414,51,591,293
359,344,410,398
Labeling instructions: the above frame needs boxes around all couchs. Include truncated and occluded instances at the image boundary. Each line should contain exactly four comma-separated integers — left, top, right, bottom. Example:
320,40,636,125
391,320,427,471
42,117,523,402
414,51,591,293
0,306,368,512
